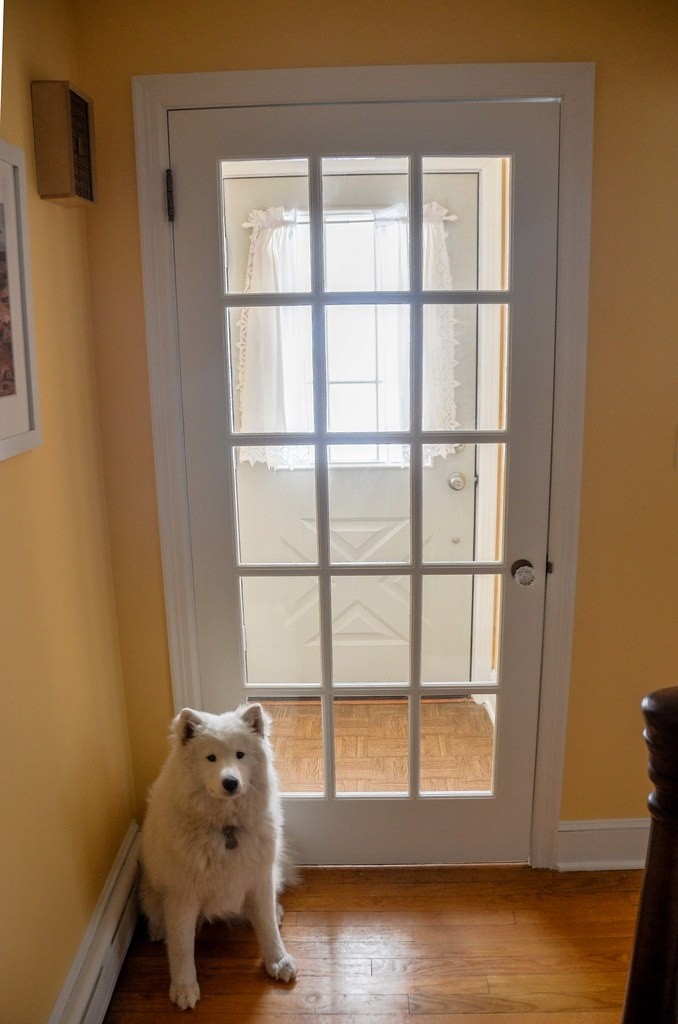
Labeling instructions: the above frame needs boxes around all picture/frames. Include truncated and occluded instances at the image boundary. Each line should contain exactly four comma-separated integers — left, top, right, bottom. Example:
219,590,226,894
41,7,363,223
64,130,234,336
0,138,41,462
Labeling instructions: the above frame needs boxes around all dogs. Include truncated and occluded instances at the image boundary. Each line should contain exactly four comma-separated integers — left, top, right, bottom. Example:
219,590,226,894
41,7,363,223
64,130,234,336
137,704,300,1010
